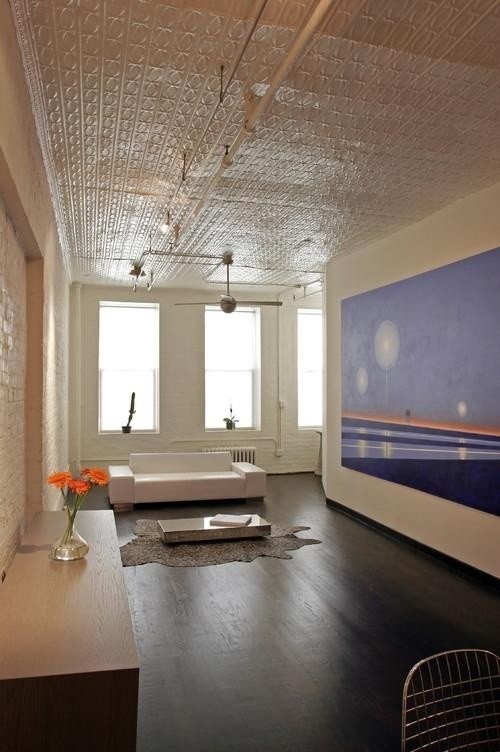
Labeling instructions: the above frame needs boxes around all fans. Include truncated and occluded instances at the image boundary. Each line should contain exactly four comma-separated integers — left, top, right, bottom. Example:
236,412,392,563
174,252,283,314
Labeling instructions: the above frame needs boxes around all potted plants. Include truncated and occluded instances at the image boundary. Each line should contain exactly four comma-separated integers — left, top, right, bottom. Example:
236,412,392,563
122,393,136,433
223,408,239,430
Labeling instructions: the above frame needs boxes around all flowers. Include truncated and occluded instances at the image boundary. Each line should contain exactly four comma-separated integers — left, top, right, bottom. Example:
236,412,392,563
48,466,110,547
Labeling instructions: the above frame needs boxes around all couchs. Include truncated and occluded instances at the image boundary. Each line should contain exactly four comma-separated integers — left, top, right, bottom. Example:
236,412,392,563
108,453,268,512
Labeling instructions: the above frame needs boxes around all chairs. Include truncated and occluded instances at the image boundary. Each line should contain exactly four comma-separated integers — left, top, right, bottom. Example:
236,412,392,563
401,649,500,752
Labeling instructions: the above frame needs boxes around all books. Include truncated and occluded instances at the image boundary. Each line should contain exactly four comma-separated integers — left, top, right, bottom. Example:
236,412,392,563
210,512,253,527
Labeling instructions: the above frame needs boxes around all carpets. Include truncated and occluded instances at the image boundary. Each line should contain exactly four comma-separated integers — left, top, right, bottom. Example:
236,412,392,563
119,517,324,568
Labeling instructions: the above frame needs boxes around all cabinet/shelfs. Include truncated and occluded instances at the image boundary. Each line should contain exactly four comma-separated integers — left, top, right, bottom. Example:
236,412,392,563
0,509,140,751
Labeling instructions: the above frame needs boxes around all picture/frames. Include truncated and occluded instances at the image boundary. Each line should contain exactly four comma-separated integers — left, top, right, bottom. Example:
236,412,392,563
54,506,89,562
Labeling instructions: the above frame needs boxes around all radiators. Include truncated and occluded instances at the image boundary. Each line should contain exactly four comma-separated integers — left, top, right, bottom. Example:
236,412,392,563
201,447,256,465
